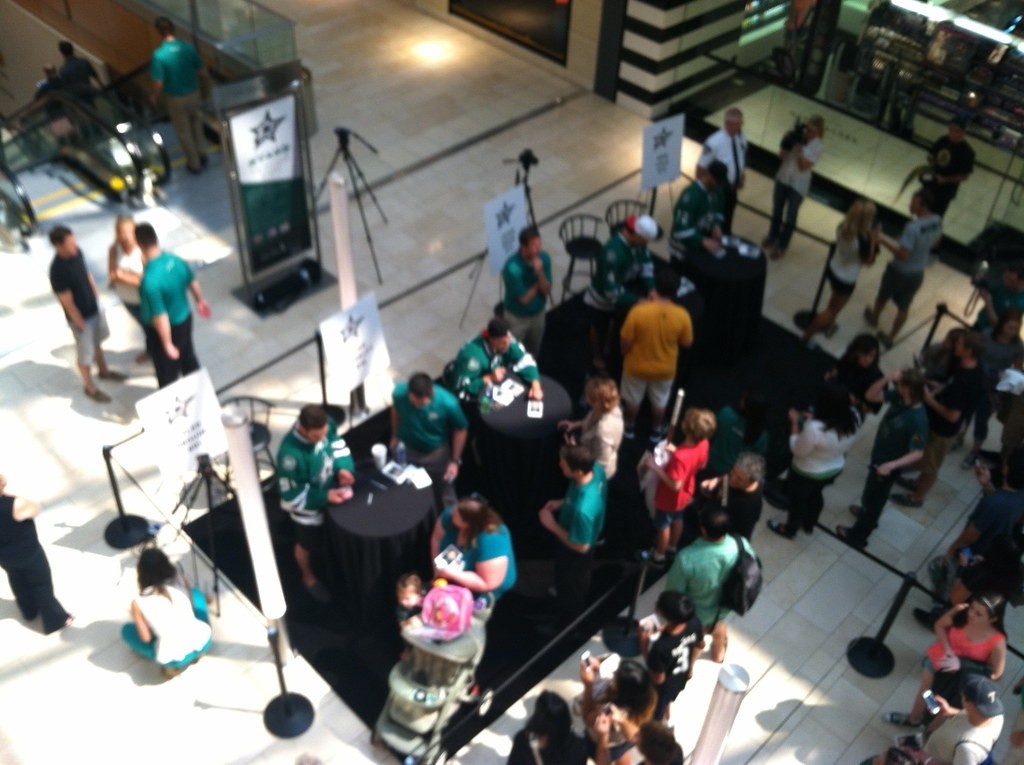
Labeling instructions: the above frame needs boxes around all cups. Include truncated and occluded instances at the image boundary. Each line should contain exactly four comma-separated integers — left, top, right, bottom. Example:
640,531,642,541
371,443,388,470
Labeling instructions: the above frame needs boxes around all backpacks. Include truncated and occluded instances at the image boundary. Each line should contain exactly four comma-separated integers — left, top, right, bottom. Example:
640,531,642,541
721,533,762,616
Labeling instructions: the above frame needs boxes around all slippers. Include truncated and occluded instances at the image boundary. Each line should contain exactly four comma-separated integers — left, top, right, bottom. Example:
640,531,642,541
712,636,727,663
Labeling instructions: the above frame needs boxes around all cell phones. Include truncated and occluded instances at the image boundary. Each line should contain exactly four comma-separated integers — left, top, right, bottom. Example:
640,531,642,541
922,689,941,714
581,650,591,672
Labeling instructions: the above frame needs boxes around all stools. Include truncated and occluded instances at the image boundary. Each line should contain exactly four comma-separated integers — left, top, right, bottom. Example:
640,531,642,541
217,394,278,495
605,198,651,239
557,213,604,303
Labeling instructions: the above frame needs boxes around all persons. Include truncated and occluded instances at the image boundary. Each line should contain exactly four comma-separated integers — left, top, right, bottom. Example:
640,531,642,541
117,547,212,678
45,14,228,402
267,0,1024,765
0,476,74,635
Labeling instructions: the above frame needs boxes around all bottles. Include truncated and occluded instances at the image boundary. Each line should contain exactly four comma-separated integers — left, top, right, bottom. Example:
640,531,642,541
485,383,494,405
480,395,490,416
396,441,407,466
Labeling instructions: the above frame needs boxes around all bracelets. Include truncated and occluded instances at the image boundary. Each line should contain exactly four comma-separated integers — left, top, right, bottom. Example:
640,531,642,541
924,755,934,765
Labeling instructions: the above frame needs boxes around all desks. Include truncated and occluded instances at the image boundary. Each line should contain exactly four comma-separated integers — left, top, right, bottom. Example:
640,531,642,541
327,463,433,594
631,268,705,378
478,372,575,499
684,233,770,361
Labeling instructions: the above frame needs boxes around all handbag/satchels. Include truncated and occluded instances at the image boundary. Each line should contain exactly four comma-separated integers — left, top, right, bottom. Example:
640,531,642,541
422,585,474,632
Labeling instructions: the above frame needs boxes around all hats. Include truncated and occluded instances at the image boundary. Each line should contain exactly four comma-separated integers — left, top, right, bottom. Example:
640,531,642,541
709,159,728,179
965,675,1004,717
628,215,657,239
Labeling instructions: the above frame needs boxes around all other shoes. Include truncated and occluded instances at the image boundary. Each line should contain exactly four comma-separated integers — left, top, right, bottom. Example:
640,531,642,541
85,388,111,401
898,476,916,491
865,308,876,327
879,332,891,349
642,551,664,568
851,505,860,514
893,493,920,506
951,441,964,452
915,608,930,628
768,520,790,537
961,451,978,468
100,372,124,382
838,526,848,538
884,712,922,727
309,583,323,601
764,238,781,259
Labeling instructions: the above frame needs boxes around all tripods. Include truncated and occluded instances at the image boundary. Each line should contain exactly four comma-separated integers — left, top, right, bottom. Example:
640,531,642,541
315,128,387,287
163,454,240,618
458,148,556,330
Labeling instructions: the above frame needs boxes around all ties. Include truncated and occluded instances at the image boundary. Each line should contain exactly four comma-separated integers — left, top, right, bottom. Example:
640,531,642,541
732,138,739,187
